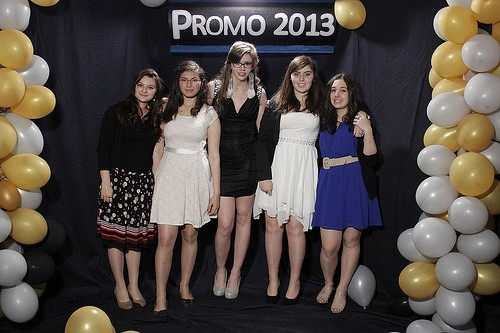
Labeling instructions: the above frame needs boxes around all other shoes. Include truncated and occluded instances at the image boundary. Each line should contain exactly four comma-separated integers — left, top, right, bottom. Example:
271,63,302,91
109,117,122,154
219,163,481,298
179,282,193,306
154,299,168,321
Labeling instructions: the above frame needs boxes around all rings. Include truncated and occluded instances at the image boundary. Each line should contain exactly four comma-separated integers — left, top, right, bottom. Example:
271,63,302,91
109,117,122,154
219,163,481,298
359,132,363,135
109,197,112,198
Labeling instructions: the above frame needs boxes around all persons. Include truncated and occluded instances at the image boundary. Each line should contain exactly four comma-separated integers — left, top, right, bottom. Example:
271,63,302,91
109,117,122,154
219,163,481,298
253,56,367,305
98,68,164,310
149,61,221,317
311,74,381,313
205,43,267,299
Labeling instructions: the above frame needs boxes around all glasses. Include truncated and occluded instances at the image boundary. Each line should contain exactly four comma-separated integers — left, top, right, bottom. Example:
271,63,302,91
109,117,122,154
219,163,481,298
179,78,201,85
233,62,254,69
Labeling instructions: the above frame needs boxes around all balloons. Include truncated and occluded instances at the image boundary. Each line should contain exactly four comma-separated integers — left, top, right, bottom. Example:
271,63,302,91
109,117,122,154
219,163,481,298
31,0,59,6
0,0,56,321
65,306,115,333
140,0,166,7
397,0,500,333
348,265,376,309
334,0,366,29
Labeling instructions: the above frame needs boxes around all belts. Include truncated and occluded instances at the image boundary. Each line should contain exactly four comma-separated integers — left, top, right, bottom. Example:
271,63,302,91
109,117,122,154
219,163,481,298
322,156,359,169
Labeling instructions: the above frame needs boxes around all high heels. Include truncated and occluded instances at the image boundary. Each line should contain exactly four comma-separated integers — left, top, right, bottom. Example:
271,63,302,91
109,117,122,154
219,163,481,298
126,284,146,306
316,284,335,303
284,283,300,304
212,269,227,296
113,288,131,309
266,279,280,304
225,275,241,299
331,291,346,313
